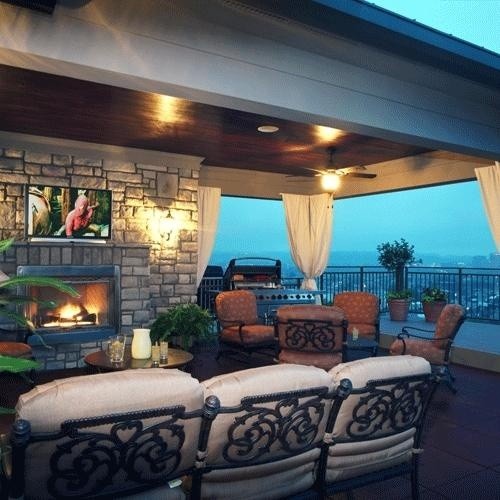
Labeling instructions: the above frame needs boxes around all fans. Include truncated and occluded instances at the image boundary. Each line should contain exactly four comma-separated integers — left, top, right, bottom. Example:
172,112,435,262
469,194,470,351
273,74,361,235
286,148,378,180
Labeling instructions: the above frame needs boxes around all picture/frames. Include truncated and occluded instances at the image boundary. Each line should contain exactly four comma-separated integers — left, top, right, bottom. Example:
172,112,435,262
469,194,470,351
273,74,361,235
24,183,112,240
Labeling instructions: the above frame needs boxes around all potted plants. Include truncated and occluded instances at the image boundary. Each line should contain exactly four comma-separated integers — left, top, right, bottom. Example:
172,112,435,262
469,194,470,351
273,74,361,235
387,291,415,320
421,288,446,322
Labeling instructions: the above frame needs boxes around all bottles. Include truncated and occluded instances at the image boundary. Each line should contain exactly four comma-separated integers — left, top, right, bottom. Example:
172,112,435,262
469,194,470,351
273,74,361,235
131,329,151,359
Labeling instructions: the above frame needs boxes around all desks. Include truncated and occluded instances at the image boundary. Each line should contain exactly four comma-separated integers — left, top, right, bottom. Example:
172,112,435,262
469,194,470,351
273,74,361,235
84,345,194,369
347,335,378,357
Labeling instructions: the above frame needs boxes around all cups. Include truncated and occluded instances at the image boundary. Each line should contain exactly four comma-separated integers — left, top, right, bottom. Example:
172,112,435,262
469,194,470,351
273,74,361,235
151,339,168,368
105,335,127,363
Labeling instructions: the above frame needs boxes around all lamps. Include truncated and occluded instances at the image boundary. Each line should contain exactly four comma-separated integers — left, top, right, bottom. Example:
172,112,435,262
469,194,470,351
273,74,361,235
321,174,338,192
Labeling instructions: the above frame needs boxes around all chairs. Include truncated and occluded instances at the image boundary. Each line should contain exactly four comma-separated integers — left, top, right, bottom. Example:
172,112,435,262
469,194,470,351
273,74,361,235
267,305,349,368
317,354,447,500
215,291,274,365
0,368,203,500
182,364,332,500
389,304,466,395
334,291,380,356
